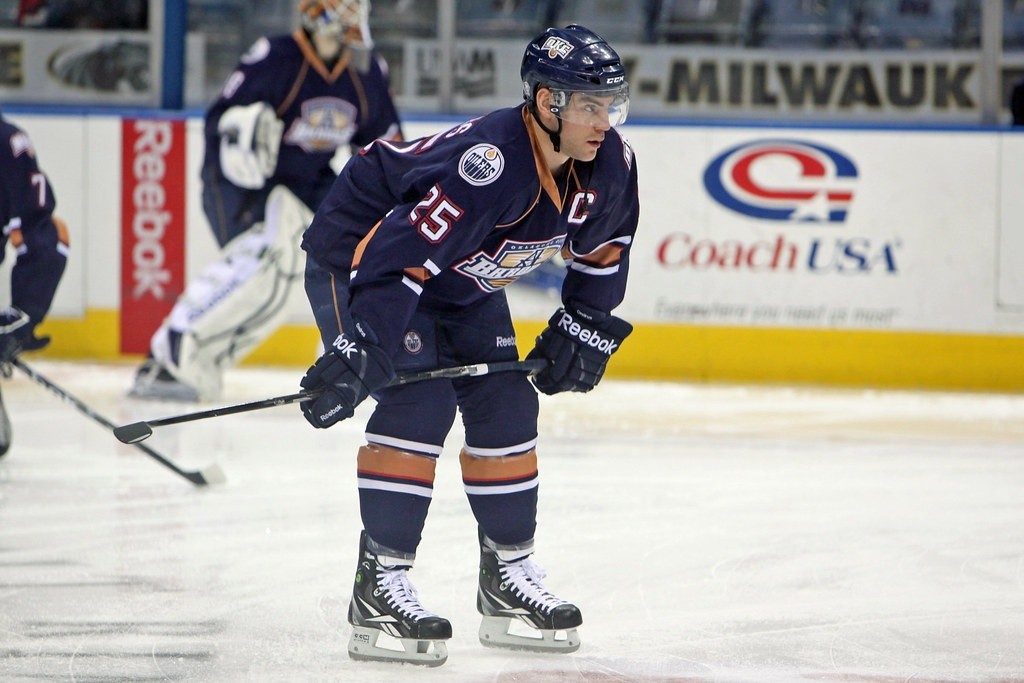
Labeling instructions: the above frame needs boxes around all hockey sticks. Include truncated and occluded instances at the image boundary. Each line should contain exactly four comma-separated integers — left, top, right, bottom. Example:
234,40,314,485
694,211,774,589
113,357,555,445
11,356,228,487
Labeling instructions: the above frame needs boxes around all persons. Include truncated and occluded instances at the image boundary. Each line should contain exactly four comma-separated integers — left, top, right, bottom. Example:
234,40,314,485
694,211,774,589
130,0,404,400
299,24,640,666
0,112,70,458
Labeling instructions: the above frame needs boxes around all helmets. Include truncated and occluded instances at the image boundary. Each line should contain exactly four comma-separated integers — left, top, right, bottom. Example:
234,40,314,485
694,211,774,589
520,23,630,127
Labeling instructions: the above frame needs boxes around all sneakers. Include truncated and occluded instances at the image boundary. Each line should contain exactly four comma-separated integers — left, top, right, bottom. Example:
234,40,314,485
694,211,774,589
348,530,453,664
476,527,582,653
129,323,236,400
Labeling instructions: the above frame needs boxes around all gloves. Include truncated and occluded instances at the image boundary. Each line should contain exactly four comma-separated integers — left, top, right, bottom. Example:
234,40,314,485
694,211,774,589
2,313,51,352
525,297,633,395
299,314,395,429
